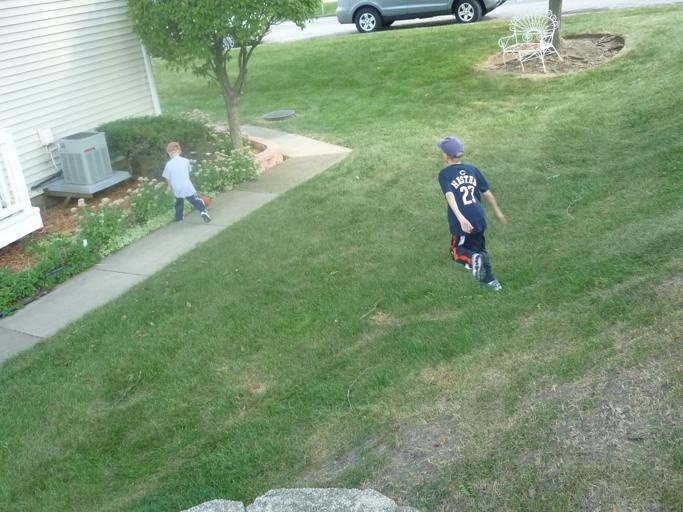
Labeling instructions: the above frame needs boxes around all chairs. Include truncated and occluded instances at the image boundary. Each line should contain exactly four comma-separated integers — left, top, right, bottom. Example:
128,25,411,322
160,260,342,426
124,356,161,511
498,7,564,74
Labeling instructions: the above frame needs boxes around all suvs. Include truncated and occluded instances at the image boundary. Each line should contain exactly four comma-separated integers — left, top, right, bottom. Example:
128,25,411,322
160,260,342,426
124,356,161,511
335,0,504,33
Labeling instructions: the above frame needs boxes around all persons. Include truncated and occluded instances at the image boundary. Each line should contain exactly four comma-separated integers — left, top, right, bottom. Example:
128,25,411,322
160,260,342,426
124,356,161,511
161,141,213,224
432,136,508,293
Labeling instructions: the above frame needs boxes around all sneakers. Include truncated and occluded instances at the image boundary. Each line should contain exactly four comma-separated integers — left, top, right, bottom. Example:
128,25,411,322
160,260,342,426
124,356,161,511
200,211,211,224
489,279,502,291
471,253,486,283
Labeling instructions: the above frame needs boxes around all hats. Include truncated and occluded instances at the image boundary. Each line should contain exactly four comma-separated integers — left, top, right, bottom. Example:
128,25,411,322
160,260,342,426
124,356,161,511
437,137,465,158
